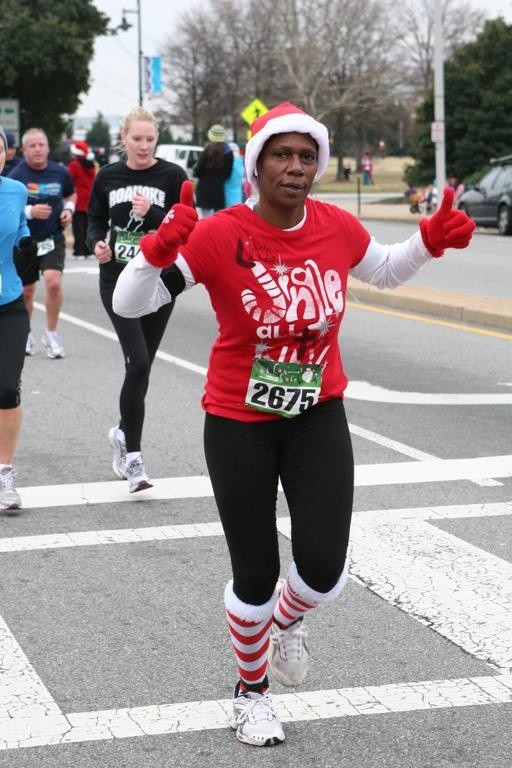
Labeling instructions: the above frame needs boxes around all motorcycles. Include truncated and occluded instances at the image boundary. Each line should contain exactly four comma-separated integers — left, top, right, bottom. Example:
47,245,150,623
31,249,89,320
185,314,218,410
408,195,431,215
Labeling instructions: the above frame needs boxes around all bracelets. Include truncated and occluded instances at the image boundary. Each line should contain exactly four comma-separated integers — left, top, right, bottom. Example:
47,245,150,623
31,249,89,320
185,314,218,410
62,200,78,217
24,202,34,221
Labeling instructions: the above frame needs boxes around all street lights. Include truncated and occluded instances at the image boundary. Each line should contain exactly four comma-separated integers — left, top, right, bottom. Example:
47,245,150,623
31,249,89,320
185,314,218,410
118,1,145,109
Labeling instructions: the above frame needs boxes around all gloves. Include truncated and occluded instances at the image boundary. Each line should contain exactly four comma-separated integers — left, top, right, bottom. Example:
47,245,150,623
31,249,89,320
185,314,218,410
417,184,474,260
142,180,204,270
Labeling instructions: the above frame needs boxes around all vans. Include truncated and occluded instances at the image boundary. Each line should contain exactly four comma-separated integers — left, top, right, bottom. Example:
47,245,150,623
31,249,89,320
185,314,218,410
154,143,204,180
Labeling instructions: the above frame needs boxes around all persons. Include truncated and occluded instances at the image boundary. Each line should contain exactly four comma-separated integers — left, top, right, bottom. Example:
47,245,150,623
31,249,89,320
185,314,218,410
2,118,39,515
3,124,79,359
109,98,478,746
85,107,198,493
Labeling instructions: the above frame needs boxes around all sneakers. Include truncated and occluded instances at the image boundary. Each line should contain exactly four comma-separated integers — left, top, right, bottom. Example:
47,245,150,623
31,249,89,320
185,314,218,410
24,332,36,356
0,467,24,511
41,328,66,358
271,579,314,687
232,677,289,748
70,253,97,262
108,424,154,493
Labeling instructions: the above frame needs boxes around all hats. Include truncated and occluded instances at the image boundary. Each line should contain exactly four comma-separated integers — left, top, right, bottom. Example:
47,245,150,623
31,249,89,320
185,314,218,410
244,99,330,188
68,141,98,160
207,123,226,143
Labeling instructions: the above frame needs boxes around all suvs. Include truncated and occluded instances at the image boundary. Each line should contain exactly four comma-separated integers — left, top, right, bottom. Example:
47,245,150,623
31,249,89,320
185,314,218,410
455,156,512,234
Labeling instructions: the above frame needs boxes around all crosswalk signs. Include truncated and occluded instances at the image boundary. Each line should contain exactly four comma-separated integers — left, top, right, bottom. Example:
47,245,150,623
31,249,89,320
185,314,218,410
238,97,270,128
